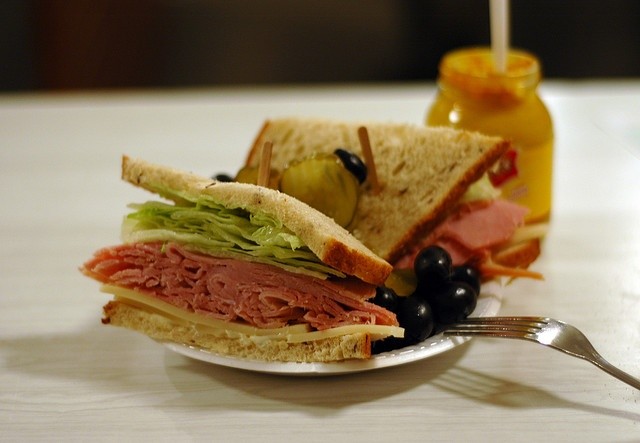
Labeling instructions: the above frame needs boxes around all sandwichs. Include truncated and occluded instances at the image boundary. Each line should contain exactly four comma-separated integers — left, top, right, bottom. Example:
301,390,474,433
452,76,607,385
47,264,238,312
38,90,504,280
235,120,508,266
79,155,403,362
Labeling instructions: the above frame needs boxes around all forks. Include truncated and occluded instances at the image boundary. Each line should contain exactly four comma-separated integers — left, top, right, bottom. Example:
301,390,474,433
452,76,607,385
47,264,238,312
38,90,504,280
442,314,640,390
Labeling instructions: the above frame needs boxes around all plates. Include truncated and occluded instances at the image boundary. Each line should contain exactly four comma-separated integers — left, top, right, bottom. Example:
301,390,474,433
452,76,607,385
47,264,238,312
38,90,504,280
158,275,502,379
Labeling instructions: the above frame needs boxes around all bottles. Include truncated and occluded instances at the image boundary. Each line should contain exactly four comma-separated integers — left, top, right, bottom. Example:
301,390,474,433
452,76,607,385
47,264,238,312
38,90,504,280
423,48,556,271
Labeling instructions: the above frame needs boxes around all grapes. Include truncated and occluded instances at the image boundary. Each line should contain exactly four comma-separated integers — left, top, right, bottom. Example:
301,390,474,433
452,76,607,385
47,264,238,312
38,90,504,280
403,245,479,349
368,286,399,313
